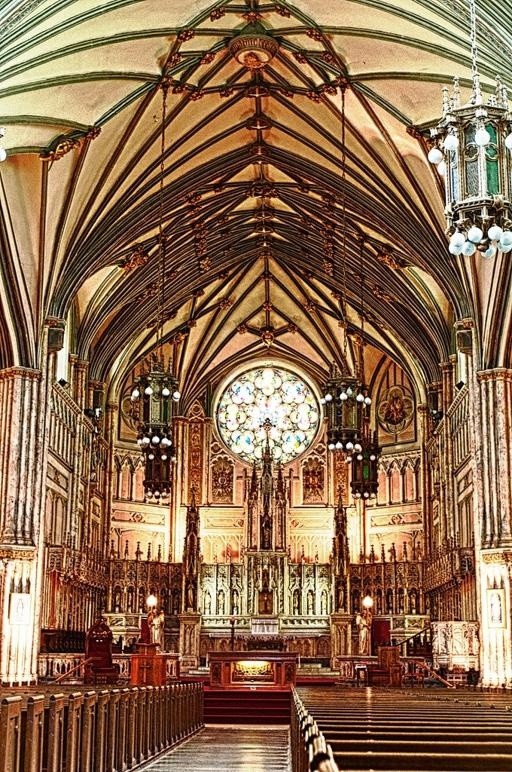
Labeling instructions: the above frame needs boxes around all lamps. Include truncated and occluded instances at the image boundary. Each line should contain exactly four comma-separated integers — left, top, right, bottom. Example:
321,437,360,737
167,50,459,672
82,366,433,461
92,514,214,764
348,232,380,500
318,76,370,454
133,78,180,453
399,0,511,266
138,234,177,494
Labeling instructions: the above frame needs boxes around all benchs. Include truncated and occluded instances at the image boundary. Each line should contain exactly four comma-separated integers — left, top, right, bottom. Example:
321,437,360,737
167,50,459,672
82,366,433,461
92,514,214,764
0,679,204,772
284,684,512,770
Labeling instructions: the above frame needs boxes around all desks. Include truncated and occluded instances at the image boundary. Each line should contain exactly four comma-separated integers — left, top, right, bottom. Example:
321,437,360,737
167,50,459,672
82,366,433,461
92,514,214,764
128,652,183,688
203,648,299,691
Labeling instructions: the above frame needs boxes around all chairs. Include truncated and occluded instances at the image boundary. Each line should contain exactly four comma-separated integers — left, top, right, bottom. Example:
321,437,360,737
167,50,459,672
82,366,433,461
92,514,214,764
365,645,403,689
80,620,119,687
